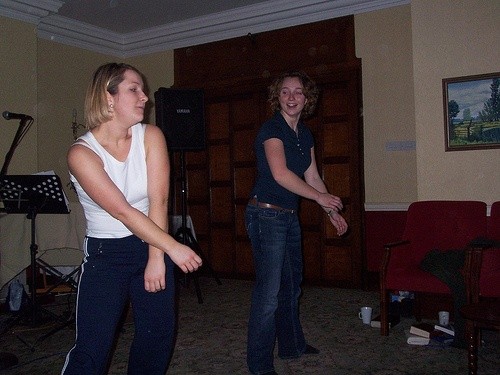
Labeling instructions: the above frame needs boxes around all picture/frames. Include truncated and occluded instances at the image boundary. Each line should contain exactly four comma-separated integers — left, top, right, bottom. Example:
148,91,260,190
441,72,500,152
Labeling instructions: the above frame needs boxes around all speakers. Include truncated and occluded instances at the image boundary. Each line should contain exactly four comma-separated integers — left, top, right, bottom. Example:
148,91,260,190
154,86,205,151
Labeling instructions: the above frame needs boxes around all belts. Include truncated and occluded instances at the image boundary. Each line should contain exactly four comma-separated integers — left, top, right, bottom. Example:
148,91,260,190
251,200,295,212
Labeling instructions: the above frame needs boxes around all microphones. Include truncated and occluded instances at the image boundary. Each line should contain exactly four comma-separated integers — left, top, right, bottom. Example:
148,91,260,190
1,111,34,121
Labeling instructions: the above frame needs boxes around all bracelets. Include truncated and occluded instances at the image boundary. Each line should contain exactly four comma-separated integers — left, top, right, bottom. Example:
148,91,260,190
327,209,334,216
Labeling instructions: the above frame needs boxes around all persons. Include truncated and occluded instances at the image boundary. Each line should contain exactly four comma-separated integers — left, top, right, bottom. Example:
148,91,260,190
244,70,348,375
59,62,202,375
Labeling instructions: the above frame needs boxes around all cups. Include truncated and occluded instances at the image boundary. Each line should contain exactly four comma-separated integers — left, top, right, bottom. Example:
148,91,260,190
439,311,449,326
358,307,372,324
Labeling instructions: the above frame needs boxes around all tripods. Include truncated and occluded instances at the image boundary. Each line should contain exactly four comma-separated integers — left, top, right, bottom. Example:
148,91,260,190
168,150,222,305
0,174,79,328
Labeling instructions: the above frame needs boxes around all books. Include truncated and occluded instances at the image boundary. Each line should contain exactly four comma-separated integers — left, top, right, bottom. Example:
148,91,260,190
405,323,455,346
369,314,399,330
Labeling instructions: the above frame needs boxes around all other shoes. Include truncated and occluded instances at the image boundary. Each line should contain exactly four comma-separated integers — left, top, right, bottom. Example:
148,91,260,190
304,345,319,355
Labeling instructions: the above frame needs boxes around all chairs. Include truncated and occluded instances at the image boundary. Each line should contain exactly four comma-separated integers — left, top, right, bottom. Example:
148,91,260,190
377,200,500,375
